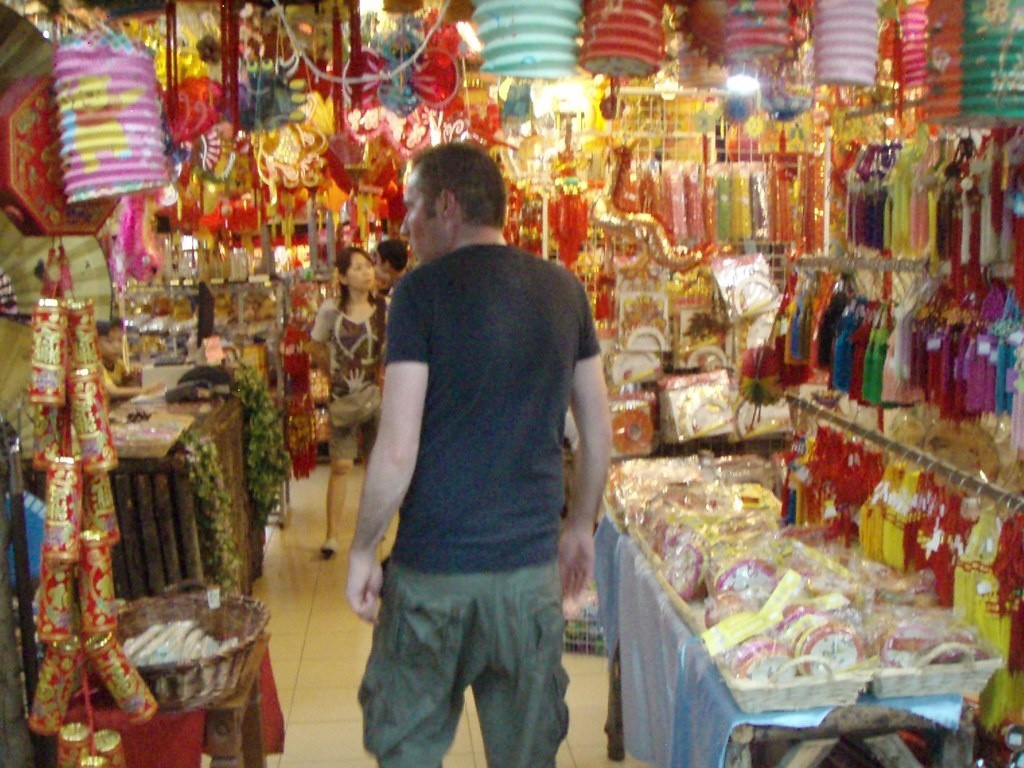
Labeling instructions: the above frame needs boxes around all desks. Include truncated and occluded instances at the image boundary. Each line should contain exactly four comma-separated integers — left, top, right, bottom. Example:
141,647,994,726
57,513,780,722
593,514,976,768
108,392,265,597
90,631,271,768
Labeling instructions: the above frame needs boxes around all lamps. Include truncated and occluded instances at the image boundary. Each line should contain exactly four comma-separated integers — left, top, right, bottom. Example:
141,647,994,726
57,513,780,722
727,64,760,95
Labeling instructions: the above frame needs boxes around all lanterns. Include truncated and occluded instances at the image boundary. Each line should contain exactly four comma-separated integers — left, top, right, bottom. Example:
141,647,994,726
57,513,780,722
470,0,583,78
901,1,1024,128
725,0,791,61
578,0,667,79
814,1,879,85
55,32,169,203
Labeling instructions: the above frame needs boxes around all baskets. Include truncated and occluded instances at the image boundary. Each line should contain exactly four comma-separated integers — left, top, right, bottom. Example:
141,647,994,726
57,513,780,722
116,594,272,712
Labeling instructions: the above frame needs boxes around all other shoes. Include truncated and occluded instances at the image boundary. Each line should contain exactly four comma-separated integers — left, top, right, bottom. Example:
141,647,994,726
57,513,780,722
321,533,337,559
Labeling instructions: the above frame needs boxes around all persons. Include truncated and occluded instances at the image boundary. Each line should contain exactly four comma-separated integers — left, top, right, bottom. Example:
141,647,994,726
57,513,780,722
349,141,612,768
310,248,389,561
377,239,409,285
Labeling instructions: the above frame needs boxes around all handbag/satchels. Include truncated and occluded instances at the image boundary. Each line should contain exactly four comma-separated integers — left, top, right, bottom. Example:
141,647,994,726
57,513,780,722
328,384,380,428
